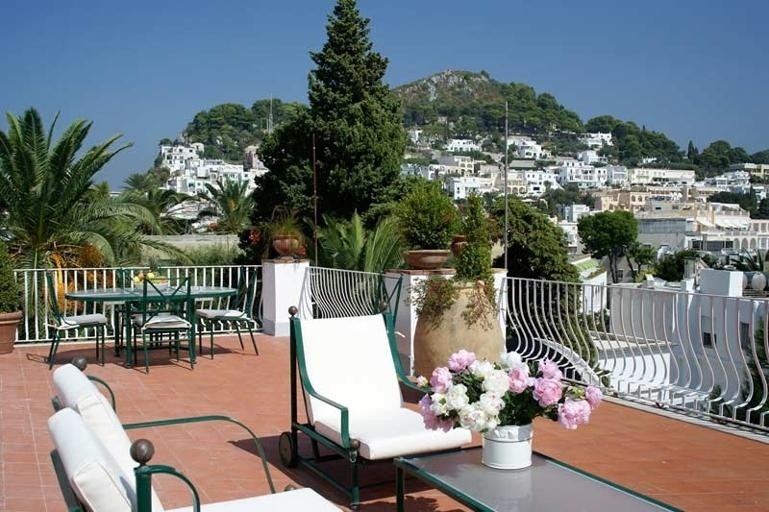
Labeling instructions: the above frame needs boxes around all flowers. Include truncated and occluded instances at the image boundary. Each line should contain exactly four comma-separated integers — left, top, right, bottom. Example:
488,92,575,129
412,344,605,434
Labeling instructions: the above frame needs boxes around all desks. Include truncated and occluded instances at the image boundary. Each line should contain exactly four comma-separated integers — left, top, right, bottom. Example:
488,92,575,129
388,438,690,512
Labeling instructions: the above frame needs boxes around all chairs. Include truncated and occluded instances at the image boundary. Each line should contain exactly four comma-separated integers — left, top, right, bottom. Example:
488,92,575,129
40,266,264,375
271,301,475,510
43,407,351,512
50,363,296,512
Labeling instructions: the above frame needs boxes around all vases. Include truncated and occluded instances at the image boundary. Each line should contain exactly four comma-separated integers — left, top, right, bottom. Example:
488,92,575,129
478,418,534,471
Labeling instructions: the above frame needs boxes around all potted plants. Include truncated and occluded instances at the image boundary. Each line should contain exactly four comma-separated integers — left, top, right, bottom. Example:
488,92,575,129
0,237,25,356
259,207,307,262
383,177,502,275
